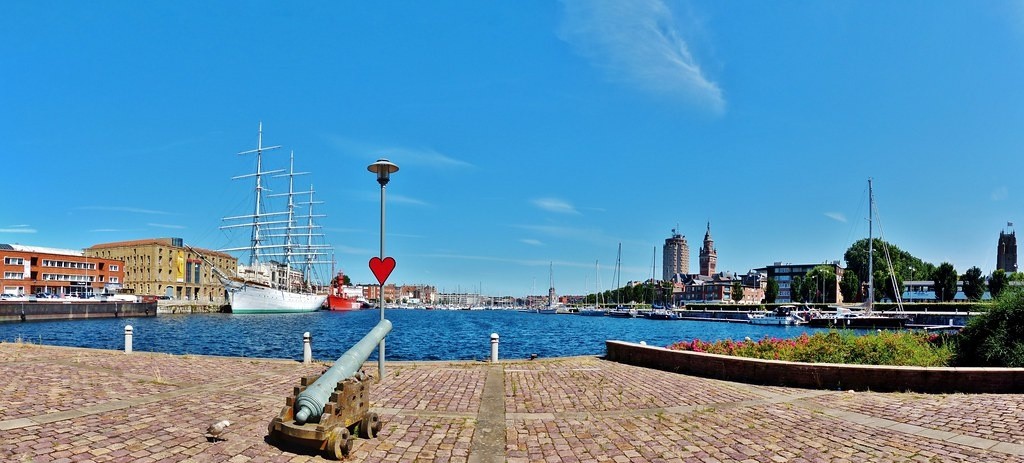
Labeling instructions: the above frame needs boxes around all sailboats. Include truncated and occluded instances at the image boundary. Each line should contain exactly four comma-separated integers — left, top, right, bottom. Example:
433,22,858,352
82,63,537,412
183,120,337,314
372,242,678,321
809,176,913,328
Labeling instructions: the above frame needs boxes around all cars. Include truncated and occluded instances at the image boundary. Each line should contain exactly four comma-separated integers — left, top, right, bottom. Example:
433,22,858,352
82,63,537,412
1,293,83,301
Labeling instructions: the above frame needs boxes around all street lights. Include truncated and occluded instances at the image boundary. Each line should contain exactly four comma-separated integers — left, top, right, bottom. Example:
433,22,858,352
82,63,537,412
81,247,88,299
366,158,400,382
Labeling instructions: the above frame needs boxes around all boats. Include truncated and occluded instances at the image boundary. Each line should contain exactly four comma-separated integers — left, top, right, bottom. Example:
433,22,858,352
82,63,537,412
746,305,799,326
327,268,362,311
